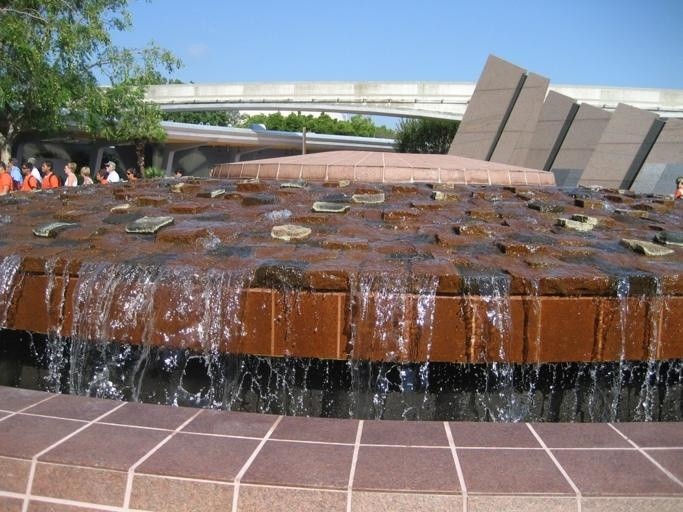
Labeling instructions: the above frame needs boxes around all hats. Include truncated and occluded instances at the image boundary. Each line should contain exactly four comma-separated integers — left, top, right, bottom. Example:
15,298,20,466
104,161,116,167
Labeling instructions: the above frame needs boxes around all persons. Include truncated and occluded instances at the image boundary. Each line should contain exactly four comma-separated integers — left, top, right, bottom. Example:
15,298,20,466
173,167,185,177
0,154,138,197
673,176,682,200
